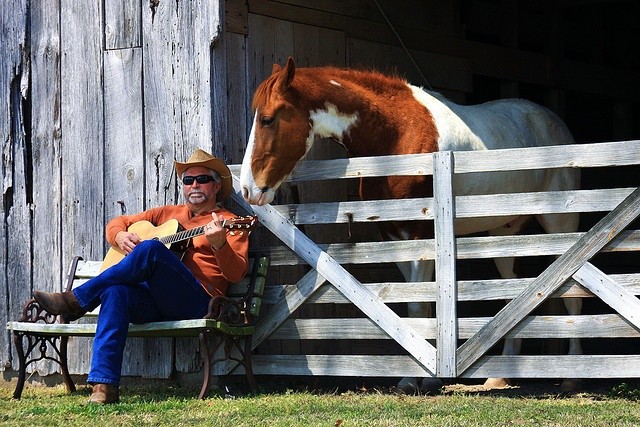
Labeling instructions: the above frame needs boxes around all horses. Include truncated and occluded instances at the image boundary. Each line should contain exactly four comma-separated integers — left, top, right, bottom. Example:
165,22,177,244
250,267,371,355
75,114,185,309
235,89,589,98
238,57,583,394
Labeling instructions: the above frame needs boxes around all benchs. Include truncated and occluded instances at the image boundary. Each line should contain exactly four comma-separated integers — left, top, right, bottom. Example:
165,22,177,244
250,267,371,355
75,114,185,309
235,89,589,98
5,250,270,399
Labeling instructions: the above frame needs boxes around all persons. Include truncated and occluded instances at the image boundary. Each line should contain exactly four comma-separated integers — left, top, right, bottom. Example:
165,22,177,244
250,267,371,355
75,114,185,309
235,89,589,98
33,148,250,407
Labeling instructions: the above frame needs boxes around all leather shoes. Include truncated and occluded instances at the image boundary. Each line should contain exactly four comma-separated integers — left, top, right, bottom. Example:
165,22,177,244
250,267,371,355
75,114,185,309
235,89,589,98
87,382,118,407
33,290,86,321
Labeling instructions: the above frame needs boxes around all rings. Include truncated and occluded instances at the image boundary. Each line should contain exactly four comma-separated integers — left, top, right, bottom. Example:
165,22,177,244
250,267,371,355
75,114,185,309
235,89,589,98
207,226,212,229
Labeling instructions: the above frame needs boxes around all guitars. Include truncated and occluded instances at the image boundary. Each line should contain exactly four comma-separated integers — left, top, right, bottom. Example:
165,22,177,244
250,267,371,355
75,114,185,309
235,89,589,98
99,213,259,274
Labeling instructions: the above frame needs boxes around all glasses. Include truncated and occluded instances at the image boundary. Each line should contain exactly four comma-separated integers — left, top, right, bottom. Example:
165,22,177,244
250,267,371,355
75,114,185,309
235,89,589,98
183,175,215,185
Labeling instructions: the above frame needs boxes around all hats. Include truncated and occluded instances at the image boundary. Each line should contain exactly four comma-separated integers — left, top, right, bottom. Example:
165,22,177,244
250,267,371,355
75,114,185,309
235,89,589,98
174,149,232,202
